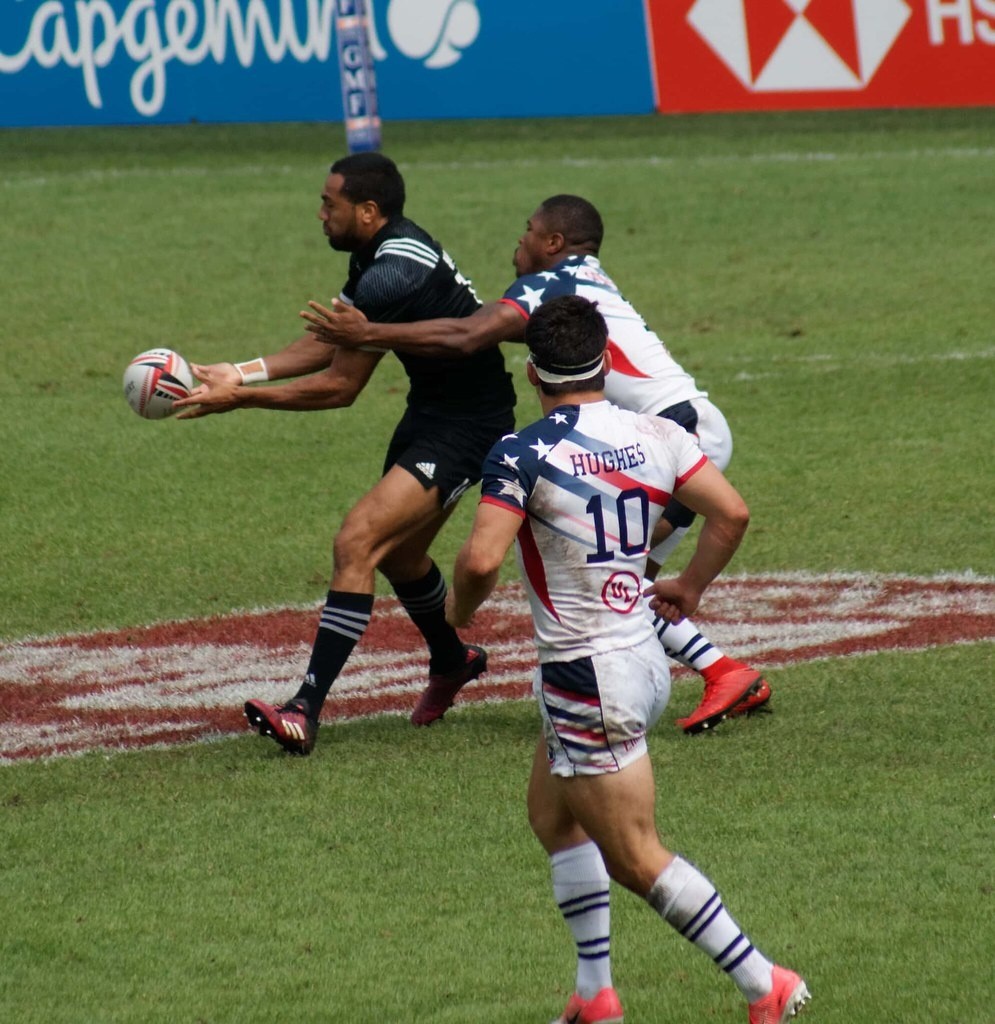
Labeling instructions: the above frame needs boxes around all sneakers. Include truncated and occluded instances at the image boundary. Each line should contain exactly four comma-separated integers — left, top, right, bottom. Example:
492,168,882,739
748,964,812,1024
556,987,623,1024
245,697,321,755
411,644,489,727
676,668,772,734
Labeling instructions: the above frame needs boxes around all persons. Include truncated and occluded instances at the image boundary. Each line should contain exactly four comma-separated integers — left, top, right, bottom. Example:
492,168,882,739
171,154,516,757
444,295,813,1024
298,194,772,735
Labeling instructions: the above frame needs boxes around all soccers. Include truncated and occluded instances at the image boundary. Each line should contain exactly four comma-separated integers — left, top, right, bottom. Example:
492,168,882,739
123,349,194,420
